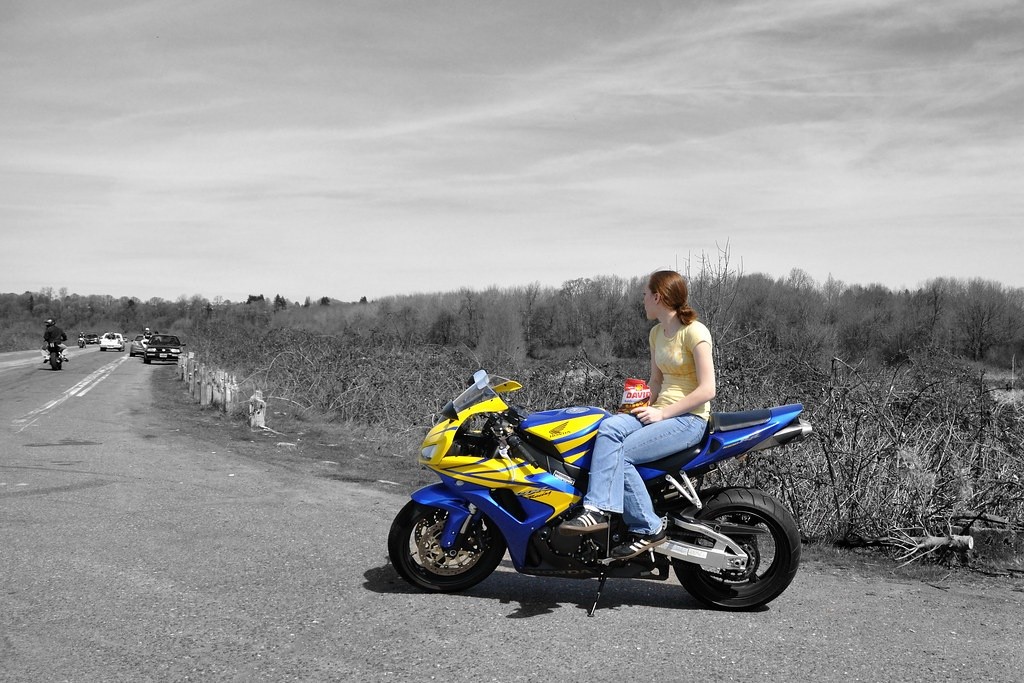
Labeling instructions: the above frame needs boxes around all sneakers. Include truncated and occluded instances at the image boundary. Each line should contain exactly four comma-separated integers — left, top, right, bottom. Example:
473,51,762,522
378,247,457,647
611,528,666,560
558,511,610,535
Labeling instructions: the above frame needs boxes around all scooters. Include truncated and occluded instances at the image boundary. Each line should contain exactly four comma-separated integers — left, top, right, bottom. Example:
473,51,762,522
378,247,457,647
78,338,85,348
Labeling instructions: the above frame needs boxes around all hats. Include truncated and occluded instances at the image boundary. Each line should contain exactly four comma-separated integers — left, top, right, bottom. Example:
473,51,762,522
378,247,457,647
145,328,149,330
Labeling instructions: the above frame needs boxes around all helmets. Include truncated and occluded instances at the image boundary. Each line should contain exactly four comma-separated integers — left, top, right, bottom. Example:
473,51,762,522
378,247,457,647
44,318,56,327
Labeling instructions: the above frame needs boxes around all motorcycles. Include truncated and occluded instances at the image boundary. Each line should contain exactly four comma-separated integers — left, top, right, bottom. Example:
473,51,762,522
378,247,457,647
43,339,66,369
384,370,814,612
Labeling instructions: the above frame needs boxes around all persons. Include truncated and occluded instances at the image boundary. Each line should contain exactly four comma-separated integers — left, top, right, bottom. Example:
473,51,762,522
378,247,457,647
143,328,153,340
78,332,86,348
170,338,175,344
152,331,162,343
40,318,69,363
559,271,715,557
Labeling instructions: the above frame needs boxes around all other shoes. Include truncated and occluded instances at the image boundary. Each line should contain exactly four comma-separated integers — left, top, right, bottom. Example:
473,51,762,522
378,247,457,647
43,359,49,364
63,356,69,362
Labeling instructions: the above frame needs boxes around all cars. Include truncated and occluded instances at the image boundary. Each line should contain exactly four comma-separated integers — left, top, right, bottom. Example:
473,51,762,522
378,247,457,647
86,333,99,344
144,334,187,364
123,336,128,342
98,332,126,352
129,334,154,357
